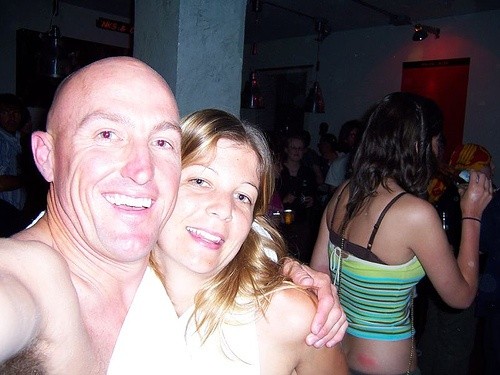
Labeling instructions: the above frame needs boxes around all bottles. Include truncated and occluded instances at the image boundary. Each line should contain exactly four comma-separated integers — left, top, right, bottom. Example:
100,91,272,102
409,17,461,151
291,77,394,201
435,167,470,189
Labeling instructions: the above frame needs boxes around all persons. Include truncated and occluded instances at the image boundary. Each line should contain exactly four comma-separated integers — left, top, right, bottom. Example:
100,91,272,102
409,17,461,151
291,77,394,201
1,56,349,374
265,118,360,260
309,91,494,374
106,108,349,375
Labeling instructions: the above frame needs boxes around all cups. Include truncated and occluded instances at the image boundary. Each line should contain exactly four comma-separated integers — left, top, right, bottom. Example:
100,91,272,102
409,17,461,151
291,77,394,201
283,209,293,224
272,210,282,225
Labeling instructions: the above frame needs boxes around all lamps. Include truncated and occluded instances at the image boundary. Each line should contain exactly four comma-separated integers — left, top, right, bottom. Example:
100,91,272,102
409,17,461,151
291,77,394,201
413,24,440,41
303,80,325,114
242,71,263,109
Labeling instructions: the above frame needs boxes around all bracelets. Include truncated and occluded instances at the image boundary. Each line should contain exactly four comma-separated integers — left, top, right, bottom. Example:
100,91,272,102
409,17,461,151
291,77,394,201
462,216,483,223
